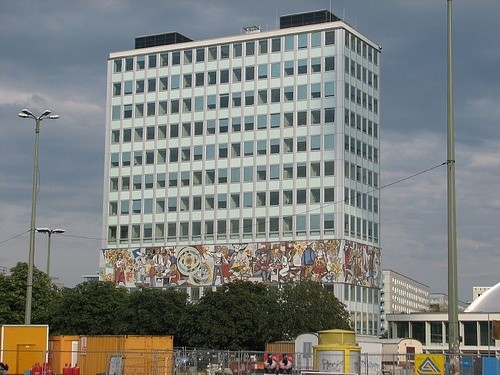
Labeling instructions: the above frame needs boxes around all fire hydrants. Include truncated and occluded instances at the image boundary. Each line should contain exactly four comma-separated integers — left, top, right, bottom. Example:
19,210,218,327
30,362,80,375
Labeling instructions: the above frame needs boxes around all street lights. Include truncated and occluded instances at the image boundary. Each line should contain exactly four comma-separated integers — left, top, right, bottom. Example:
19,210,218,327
19,104,61,326
34,224,66,282
